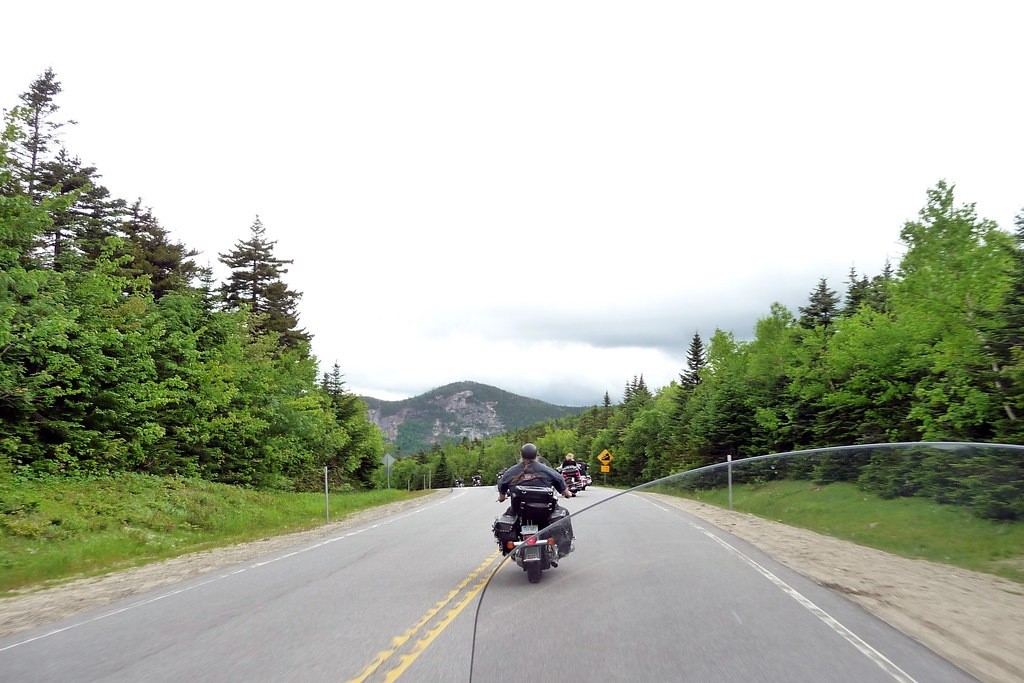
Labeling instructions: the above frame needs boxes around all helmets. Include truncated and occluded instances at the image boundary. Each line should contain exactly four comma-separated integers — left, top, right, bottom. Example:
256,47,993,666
576,458,582,462
521,443,537,460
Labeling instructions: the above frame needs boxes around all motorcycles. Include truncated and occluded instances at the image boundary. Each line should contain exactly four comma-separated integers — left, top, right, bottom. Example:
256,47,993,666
556,464,593,497
494,487,577,584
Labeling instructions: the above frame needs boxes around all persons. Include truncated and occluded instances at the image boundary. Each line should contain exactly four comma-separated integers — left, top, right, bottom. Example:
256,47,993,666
562,452,589,477
498,443,573,557
457,477,463,485
473,472,481,484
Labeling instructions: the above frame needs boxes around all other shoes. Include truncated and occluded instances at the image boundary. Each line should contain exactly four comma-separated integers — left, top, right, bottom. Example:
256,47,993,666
511,554,515,560
558,545,575,558
